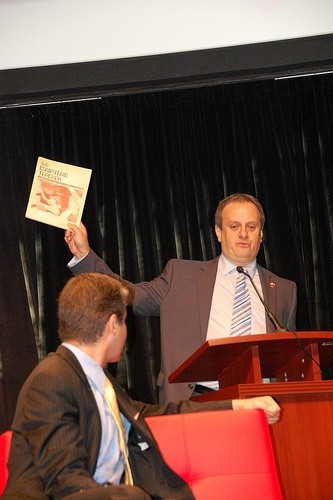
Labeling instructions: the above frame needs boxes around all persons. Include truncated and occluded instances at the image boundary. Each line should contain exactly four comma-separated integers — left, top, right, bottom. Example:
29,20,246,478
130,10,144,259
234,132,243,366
31,192,78,223
0,273,281,500
64,193,297,406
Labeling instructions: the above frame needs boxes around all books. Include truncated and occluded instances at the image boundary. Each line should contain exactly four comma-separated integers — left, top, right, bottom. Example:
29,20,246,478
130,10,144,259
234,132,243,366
26,157,92,233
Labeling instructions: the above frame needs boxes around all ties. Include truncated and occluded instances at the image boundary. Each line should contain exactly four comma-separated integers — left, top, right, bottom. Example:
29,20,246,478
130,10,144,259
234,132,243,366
229,271,253,336
102,376,135,487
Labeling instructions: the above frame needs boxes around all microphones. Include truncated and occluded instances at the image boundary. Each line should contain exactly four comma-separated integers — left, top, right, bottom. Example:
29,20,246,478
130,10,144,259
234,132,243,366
237,266,287,333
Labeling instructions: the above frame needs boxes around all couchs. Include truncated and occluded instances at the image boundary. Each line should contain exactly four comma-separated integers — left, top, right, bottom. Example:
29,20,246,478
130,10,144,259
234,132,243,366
0,409,283,500
